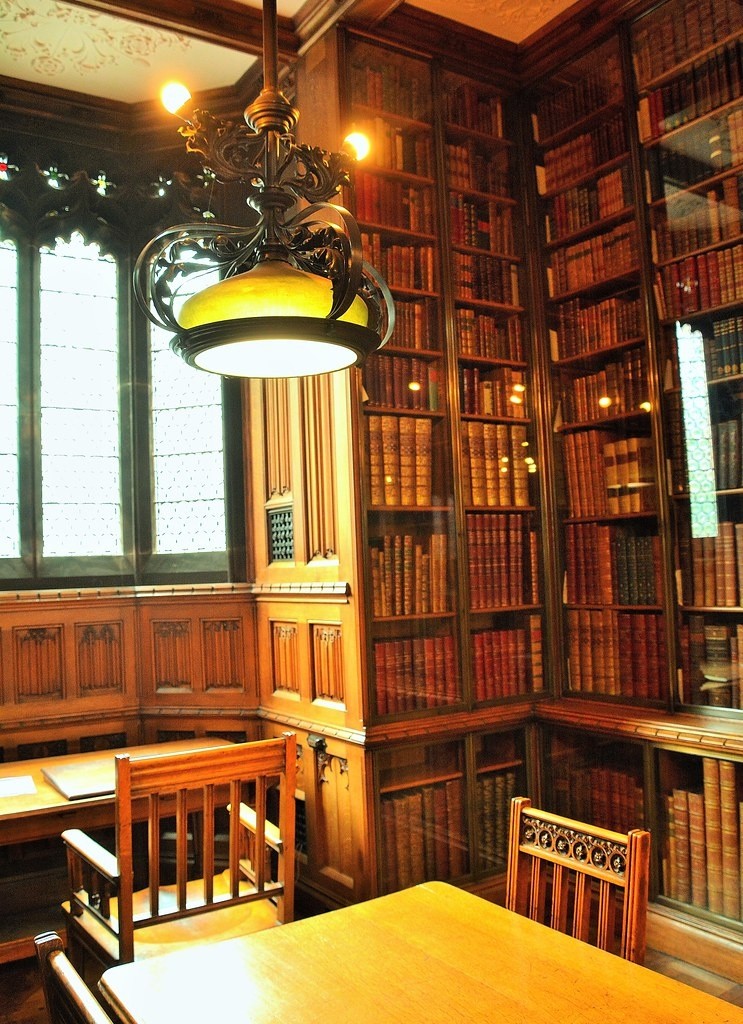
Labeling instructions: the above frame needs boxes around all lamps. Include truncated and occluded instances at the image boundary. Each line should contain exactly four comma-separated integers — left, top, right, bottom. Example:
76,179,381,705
133,0,395,378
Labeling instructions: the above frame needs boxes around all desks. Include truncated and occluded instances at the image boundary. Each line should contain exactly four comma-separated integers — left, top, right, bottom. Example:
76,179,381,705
97,880,743,1024
0,735,249,965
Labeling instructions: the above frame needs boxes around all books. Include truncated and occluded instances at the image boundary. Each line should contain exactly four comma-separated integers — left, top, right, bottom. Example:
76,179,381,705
361,233,434,292
443,83,504,138
445,144,508,198
660,616,743,923
639,0,743,83
382,773,518,893
545,113,640,297
565,765,643,838
563,430,656,518
40,760,115,800
351,61,426,120
354,171,433,234
456,308,522,362
452,251,519,304
450,193,516,257
565,523,667,699
381,298,438,351
639,35,743,201
652,176,743,608
353,117,435,178
537,53,623,142
370,514,542,715
364,354,530,506
559,297,655,423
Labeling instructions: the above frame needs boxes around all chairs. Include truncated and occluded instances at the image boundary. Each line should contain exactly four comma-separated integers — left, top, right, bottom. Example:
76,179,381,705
60,731,296,977
505,796,649,965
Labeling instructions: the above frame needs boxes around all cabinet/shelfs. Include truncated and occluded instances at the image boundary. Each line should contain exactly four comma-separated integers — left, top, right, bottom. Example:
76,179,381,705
336,0,743,987
